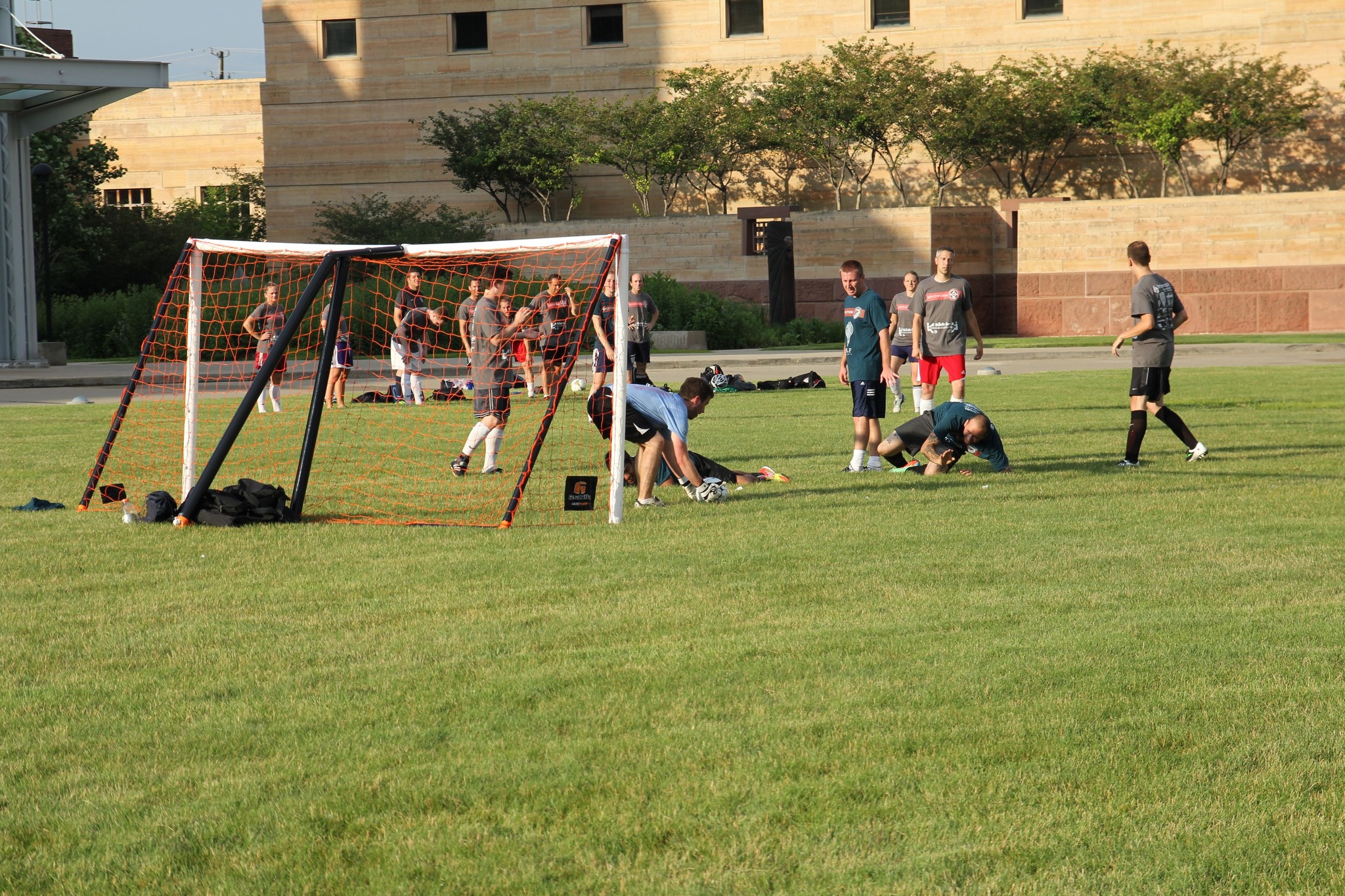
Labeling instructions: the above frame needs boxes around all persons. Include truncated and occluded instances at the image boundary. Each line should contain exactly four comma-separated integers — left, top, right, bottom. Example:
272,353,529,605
627,272,659,387
837,259,901,476
448,264,545,484
393,270,433,404
320,282,355,411
586,269,636,425
875,400,1012,484
523,272,582,400
498,298,537,399
455,276,485,374
881,270,925,415
586,376,728,513
1110,239,1210,470
390,305,450,408
905,246,984,421
606,446,794,491
242,281,289,416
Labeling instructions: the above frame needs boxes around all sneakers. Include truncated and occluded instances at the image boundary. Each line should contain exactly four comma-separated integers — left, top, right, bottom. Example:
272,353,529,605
864,466,882,472
1185,441,1208,462
892,393,904,413
543,396,550,401
841,467,864,474
480,468,503,475
758,466,790,482
450,458,468,477
1114,459,1140,467
914,407,920,413
633,496,668,509
890,459,920,473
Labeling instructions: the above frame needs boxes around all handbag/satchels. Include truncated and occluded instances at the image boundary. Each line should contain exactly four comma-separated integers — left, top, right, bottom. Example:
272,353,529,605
175,486,247,527
223,478,292,524
788,371,826,388
432,387,466,402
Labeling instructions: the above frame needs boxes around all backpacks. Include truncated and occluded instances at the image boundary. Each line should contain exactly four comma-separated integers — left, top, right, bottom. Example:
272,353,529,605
138,490,177,523
699,364,724,384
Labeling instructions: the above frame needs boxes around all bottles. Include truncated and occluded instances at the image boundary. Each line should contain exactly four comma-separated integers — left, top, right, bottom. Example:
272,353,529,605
121,498,138,524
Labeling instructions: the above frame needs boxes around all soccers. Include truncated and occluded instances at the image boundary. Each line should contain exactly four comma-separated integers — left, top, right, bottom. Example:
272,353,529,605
695,476,728,503
570,378,586,392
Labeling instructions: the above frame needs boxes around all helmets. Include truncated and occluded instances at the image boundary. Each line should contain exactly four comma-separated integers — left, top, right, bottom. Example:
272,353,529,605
711,374,728,389
